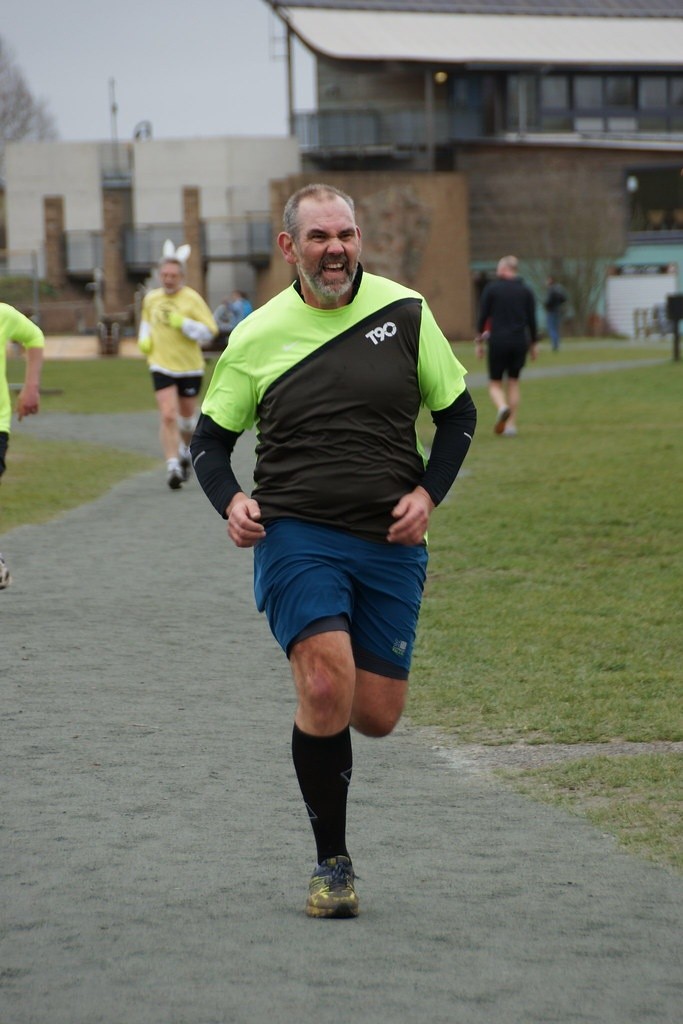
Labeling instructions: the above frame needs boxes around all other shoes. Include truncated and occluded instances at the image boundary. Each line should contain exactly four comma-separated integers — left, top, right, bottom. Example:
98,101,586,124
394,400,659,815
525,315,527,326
178,453,192,481
504,426,517,436
167,468,183,490
494,406,511,435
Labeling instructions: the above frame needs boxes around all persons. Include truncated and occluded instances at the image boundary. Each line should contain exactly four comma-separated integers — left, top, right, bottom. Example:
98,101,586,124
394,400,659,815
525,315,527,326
0,302,46,587
216,289,252,347
138,257,219,490
474,254,536,438
190,184,478,916
542,276,568,347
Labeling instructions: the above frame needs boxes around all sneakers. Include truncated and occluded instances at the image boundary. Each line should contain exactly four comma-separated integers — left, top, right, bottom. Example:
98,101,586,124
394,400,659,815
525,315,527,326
306,856,359,919
0,553,11,589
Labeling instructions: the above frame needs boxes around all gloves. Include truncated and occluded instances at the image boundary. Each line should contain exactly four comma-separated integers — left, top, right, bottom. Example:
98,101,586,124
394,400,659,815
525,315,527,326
139,338,152,352
166,312,183,330
